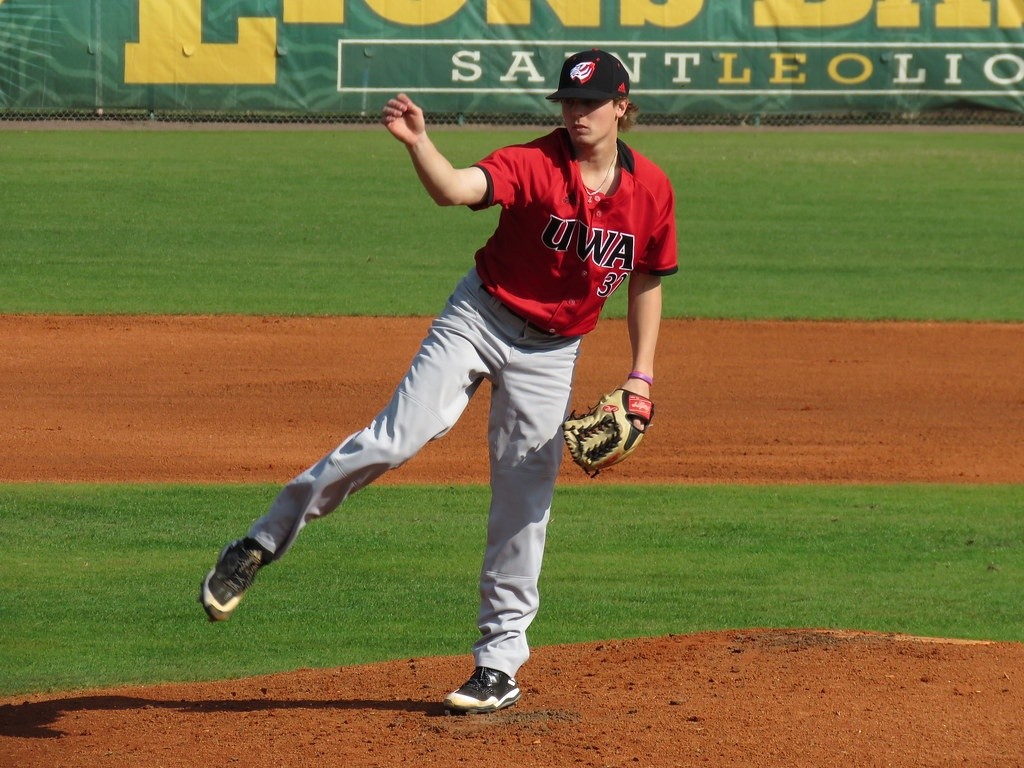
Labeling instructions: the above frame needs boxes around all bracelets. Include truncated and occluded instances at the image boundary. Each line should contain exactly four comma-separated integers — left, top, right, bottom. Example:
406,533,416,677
627,372,654,387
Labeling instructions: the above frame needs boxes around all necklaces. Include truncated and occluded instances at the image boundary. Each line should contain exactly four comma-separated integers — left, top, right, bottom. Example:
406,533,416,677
583,144,618,203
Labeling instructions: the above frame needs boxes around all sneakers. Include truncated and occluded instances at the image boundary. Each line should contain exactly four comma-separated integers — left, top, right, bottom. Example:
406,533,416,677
199,539,264,622
443,666,522,714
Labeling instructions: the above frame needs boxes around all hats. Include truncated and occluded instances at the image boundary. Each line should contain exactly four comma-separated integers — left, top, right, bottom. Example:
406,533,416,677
545,47,630,100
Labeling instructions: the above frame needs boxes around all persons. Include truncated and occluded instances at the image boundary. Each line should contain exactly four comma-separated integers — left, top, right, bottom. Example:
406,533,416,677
199,49,678,714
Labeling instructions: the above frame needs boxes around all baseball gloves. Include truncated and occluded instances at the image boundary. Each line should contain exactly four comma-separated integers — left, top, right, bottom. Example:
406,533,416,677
562,389,655,471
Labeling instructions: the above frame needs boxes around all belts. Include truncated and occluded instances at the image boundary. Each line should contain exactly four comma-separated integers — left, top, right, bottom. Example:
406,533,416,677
480,283,556,336
